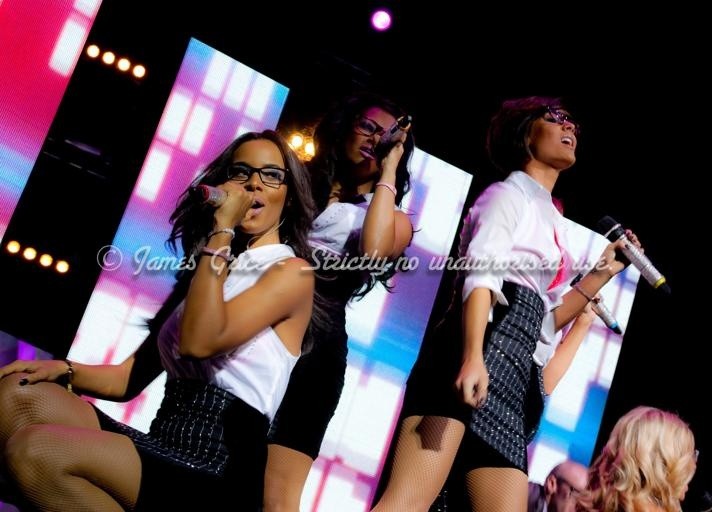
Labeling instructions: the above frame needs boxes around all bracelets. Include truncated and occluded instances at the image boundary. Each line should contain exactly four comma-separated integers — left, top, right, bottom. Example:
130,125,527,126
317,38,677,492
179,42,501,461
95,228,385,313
61,358,74,384
375,181,399,195
200,246,231,262
572,282,593,301
204,226,237,238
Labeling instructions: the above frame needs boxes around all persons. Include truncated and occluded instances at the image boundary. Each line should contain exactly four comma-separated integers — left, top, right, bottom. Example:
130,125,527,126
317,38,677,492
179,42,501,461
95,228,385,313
526,460,590,511
430,293,606,510
370,95,647,511
1,129,317,511
261,93,415,511
575,403,698,512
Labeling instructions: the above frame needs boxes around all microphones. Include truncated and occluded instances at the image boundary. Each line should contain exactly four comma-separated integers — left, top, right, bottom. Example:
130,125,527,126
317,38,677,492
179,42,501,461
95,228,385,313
569,273,623,335
189,184,227,208
598,217,666,291
373,115,413,155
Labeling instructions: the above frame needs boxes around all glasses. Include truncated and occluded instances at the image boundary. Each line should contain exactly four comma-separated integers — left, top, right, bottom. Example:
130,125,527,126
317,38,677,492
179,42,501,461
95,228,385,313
354,116,385,137
226,162,292,188
535,103,584,129
